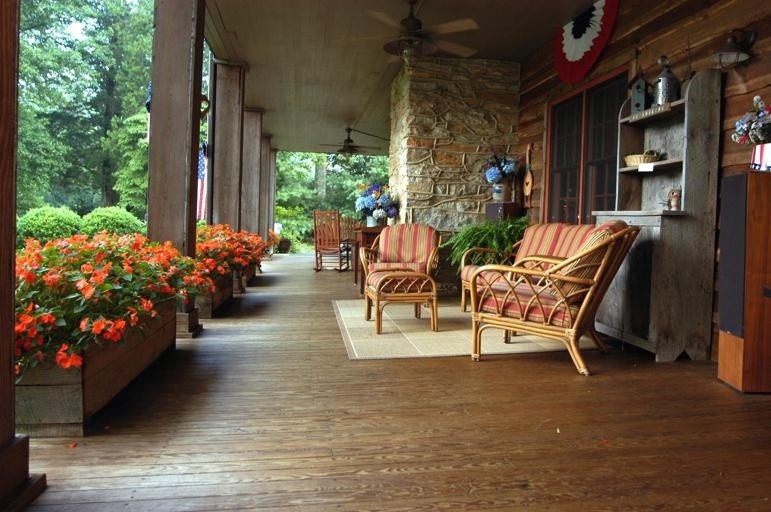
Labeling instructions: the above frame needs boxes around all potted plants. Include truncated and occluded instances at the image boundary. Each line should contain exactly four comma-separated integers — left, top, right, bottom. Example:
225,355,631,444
278,230,300,253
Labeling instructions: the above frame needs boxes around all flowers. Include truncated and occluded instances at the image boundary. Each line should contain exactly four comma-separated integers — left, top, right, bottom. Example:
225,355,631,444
354,181,399,220
732,95,771,145
485,145,517,185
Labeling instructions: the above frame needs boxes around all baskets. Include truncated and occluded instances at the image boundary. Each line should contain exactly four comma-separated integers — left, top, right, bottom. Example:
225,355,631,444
625,150,657,167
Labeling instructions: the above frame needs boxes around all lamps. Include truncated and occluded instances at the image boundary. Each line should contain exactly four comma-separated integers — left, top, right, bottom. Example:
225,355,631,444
337,147,358,157
382,36,439,68
708,28,757,70
386,217,395,226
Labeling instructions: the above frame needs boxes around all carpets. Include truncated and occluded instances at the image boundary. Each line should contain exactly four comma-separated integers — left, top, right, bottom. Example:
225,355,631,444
330,295,616,361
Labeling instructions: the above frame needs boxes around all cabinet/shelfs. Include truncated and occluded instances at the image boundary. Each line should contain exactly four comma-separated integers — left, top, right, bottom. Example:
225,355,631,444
486,203,524,219
717,171,771,394
590,68,722,364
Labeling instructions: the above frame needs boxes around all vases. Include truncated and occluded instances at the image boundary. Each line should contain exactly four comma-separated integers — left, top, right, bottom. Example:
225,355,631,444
367,215,378,228
15,296,177,438
750,142,771,172
493,181,510,202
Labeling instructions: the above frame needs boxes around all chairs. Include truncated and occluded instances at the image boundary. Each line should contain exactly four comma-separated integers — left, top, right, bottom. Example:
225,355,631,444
313,210,350,272
359,232,442,308
469,226,641,377
460,239,595,336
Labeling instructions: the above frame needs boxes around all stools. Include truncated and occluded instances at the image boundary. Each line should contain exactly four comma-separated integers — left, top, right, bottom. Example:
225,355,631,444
364,268,438,335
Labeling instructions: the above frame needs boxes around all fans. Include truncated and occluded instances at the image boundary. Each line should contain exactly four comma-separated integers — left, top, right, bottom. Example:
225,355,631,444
320,128,382,151
325,0,480,64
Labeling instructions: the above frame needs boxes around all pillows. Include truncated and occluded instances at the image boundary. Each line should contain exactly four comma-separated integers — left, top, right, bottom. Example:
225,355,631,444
378,223,439,262
551,219,628,305
514,223,595,277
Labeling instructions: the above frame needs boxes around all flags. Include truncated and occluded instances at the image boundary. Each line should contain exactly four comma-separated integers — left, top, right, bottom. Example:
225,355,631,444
196,150,207,220
